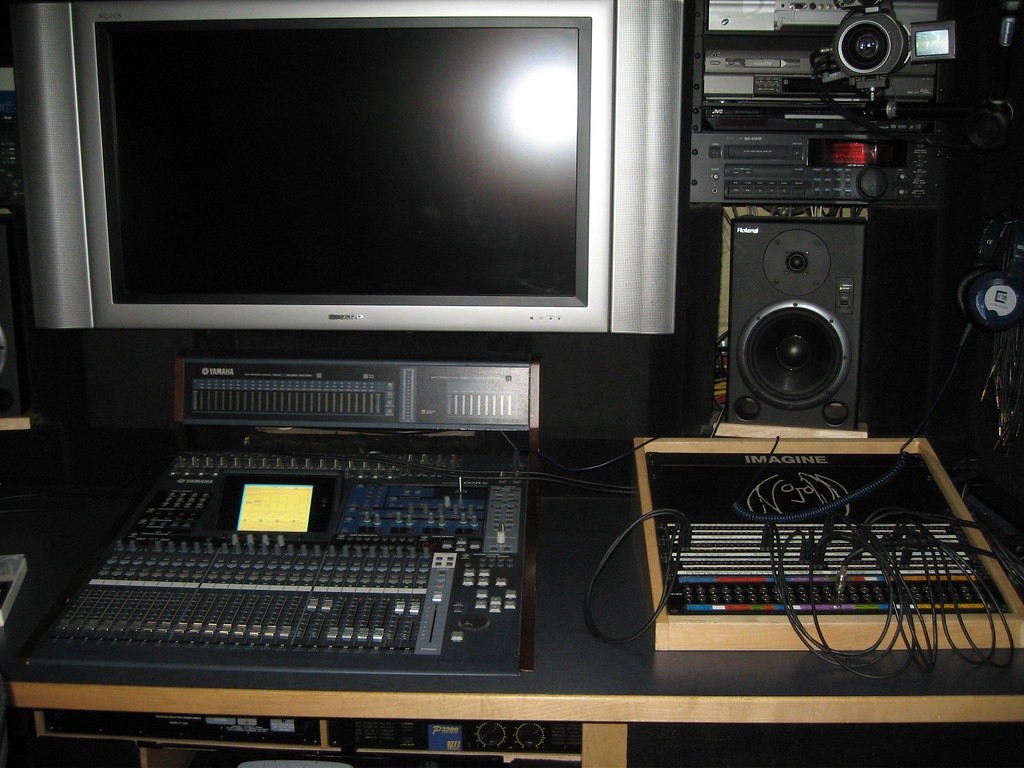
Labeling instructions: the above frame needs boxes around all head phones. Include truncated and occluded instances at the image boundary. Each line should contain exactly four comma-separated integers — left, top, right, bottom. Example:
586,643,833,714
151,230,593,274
956,212,1024,332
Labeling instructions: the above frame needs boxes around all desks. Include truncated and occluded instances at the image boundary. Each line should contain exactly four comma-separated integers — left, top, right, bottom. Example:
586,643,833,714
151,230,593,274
0,426,1024,768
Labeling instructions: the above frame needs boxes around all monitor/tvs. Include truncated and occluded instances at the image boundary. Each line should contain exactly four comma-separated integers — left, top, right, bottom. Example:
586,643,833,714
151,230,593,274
15,0,686,330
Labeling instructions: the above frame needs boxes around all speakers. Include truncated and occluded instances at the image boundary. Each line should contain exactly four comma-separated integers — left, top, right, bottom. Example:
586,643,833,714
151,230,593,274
727,213,867,431
